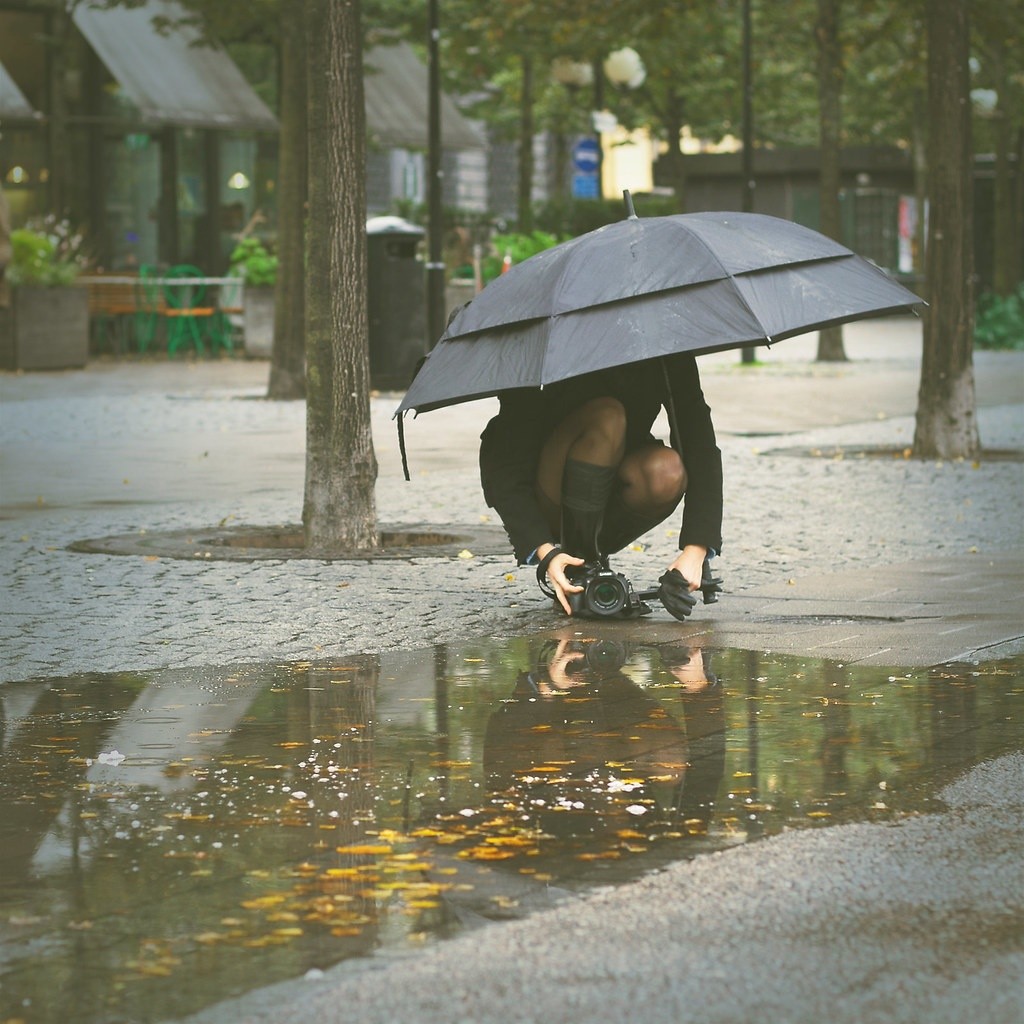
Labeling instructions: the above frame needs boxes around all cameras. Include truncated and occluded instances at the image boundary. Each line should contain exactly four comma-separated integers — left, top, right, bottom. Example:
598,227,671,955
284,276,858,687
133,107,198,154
564,564,630,616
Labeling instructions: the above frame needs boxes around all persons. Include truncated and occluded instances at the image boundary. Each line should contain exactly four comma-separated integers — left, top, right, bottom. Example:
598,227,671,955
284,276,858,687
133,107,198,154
479,350,724,622
211,200,268,276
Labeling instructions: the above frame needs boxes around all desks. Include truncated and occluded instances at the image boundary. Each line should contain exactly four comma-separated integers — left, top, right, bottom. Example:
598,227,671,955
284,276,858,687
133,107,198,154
80,275,243,354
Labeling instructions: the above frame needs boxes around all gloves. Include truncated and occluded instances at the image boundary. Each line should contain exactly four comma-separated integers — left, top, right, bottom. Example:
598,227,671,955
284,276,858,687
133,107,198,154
658,568,697,623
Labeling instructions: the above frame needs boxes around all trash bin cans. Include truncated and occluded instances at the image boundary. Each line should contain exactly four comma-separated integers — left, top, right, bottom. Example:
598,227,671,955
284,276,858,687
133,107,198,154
366,216,435,394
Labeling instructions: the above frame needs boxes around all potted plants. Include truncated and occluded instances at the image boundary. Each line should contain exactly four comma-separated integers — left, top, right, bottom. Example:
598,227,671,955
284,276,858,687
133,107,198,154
0,226,88,375
230,238,279,360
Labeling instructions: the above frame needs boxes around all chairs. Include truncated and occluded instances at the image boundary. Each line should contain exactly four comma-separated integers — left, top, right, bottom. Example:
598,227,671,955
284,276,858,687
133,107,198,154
91,263,239,359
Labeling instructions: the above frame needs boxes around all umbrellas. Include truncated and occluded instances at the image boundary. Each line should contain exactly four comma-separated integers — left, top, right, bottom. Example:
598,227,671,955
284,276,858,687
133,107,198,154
391,190,931,604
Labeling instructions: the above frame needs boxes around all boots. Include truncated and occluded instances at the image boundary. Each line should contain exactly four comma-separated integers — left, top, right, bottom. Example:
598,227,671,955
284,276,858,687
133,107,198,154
550,456,657,619
